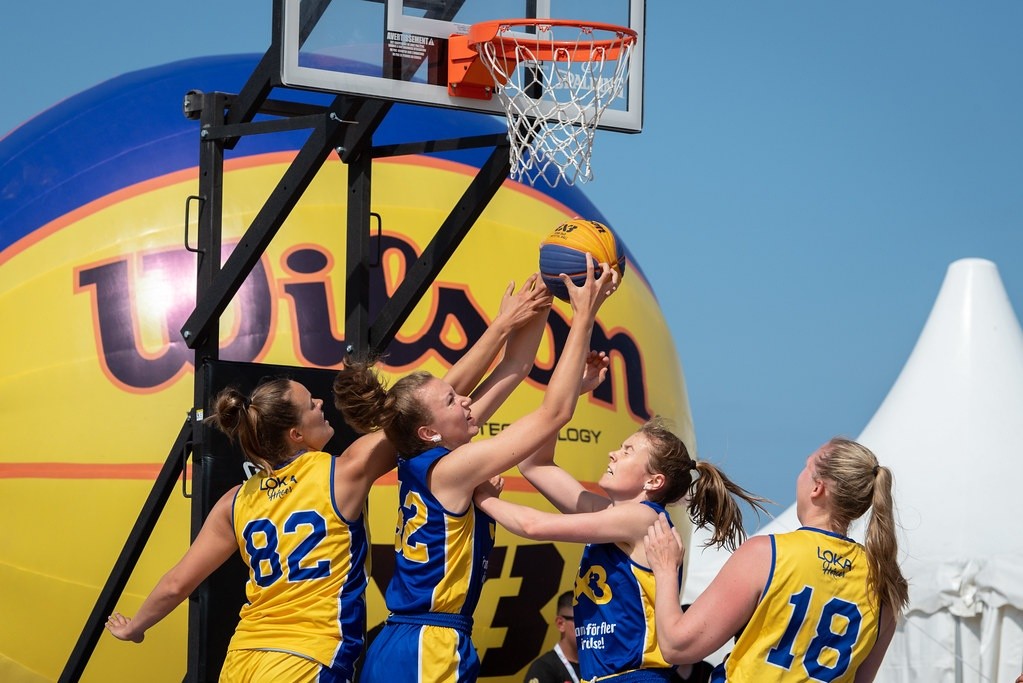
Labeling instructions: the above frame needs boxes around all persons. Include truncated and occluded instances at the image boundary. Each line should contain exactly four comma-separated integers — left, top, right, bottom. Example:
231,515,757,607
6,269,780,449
104,271,554,683
643,438,911,683
332,252,776,683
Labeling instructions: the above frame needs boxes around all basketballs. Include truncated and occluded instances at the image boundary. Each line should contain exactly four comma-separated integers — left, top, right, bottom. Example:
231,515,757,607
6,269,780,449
539,219,626,304
